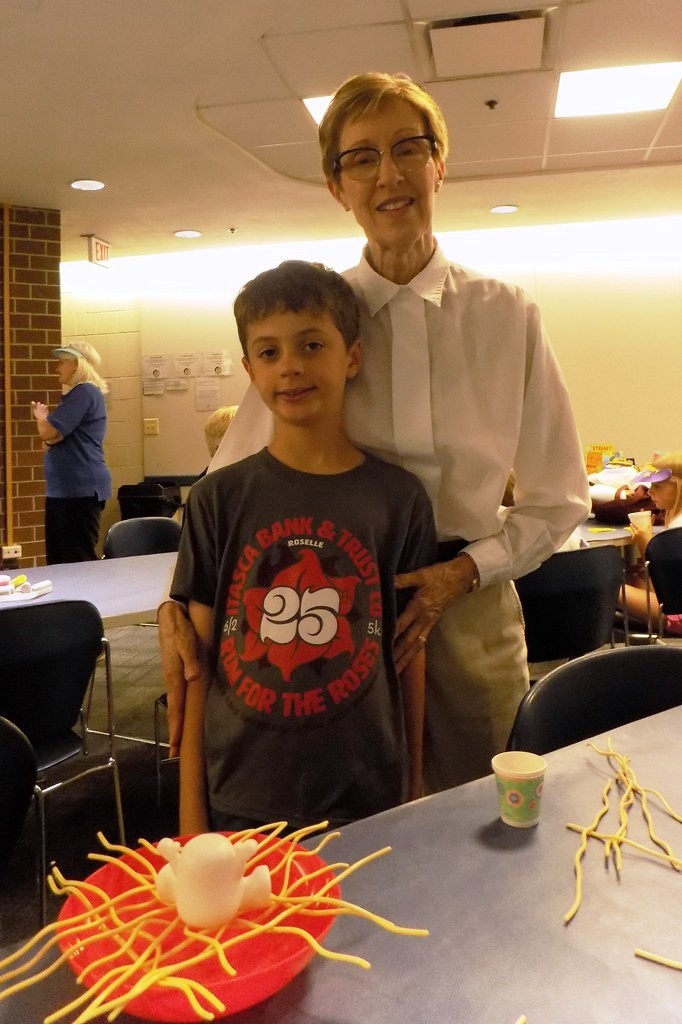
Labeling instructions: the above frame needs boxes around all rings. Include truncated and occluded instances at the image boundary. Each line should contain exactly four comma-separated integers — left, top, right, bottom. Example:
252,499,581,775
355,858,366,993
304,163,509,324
419,635,428,644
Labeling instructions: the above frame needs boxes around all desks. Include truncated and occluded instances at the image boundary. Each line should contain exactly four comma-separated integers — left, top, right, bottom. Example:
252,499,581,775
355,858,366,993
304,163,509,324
0,706,682,1024
0,552,181,629
577,518,667,646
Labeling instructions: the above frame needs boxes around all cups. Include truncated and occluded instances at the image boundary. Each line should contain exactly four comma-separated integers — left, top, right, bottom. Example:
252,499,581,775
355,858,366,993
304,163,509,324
628,511,652,535
491,750,549,830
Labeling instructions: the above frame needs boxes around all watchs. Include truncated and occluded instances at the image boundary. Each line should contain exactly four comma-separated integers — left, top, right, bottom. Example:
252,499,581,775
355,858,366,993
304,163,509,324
461,552,480,593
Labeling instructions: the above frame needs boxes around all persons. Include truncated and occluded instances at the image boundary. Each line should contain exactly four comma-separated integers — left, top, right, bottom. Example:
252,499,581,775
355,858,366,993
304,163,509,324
198,405,240,477
179,262,438,840
29,342,113,566
618,452,682,638
155,73,592,800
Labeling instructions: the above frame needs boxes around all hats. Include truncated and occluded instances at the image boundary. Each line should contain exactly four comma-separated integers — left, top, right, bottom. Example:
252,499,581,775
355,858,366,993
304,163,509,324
52,348,80,358
629,466,673,484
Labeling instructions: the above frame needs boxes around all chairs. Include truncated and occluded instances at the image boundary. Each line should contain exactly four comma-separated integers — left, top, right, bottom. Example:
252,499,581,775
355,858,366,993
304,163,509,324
507,649,682,755
0,600,127,927
102,516,182,560
613,527,682,648
0,716,39,877
154,692,181,807
513,544,626,688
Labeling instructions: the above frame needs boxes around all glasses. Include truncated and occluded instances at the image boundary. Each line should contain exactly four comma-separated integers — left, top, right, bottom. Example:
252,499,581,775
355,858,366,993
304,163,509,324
332,135,437,181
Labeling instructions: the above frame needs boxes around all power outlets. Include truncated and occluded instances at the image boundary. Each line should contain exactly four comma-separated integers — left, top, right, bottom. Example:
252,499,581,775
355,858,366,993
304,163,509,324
1,545,23,559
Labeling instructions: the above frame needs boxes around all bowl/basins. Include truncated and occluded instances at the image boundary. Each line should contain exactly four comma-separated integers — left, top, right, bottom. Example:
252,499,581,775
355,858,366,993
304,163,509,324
54,832,341,1022
